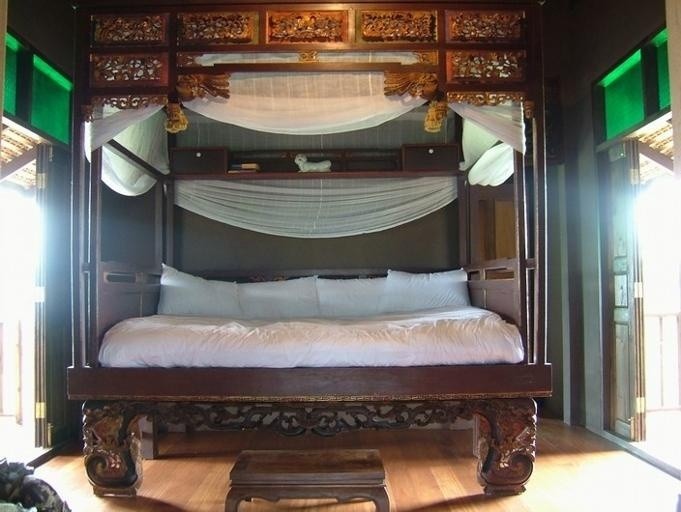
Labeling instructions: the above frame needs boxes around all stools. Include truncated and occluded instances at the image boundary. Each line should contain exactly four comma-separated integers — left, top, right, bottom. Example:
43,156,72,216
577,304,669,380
226,446,394,511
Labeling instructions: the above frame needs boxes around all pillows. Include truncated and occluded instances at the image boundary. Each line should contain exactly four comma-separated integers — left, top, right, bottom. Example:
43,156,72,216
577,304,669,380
155,262,245,317
232,276,323,319
314,272,388,316
385,266,474,314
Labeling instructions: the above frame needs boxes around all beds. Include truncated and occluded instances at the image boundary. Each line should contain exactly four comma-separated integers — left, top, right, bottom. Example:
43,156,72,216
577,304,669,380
63,78,553,497
